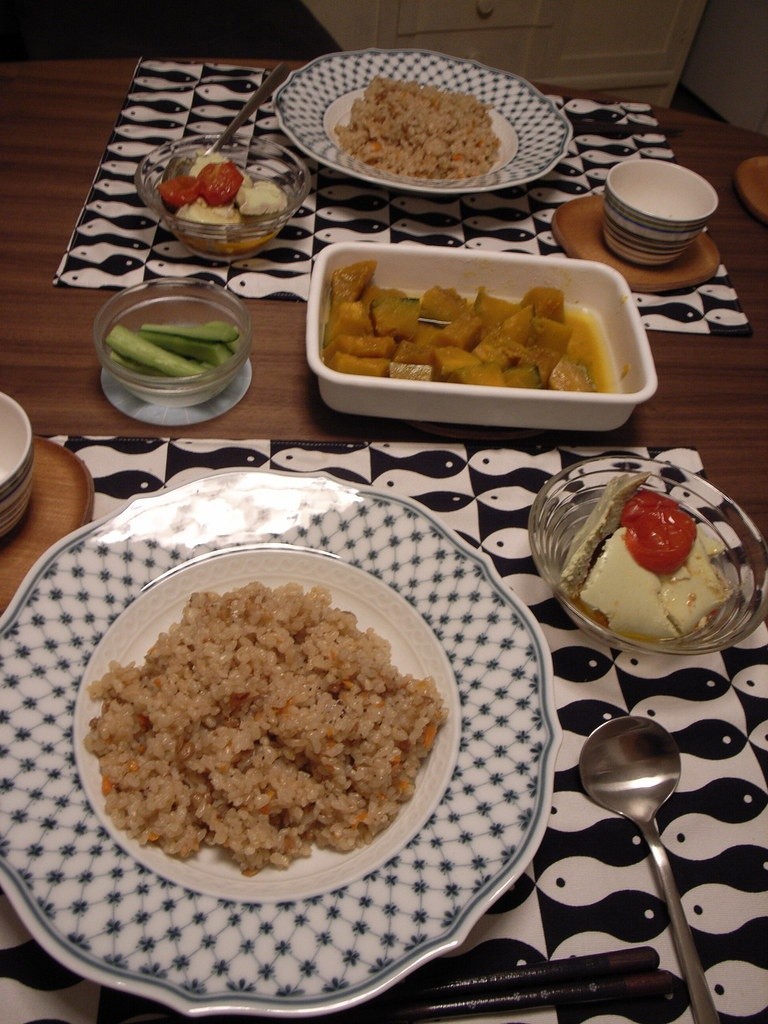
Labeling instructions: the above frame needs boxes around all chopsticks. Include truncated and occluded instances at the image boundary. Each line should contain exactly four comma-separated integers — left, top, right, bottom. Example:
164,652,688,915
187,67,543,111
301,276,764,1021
317,945,673,1023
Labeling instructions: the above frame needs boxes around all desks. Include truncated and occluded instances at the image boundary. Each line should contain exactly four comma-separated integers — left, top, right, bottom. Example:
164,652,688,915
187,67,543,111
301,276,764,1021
0,57,768,1024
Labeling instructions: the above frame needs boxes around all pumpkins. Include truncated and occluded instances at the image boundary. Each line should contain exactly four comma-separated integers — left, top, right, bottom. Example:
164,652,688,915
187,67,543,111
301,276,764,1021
321,259,600,394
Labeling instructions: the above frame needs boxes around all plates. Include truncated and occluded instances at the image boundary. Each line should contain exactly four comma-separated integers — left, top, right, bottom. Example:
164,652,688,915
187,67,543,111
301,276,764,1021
1,464,558,1019
272,47,574,192
99,359,253,426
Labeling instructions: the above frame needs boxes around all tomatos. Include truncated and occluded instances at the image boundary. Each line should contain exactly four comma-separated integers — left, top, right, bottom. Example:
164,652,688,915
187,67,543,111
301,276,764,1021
157,174,202,207
618,490,697,575
196,161,243,206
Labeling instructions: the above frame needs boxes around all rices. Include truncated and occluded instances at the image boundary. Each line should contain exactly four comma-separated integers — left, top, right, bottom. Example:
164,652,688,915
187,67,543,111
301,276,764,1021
333,75,501,179
83,581,451,878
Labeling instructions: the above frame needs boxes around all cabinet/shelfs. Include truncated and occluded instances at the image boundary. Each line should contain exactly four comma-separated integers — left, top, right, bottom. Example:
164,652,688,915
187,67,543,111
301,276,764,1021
300,0,706,108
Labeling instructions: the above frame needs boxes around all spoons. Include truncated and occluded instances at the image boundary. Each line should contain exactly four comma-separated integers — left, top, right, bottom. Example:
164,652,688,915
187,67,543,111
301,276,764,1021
580,717,723,1023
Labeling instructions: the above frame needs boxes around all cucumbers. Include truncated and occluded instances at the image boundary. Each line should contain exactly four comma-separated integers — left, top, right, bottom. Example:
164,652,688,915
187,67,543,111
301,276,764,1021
105,322,241,380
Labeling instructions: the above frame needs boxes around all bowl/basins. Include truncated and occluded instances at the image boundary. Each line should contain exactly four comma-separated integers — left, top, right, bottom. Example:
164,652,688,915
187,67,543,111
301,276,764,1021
528,457,767,659
93,277,252,407
305,242,658,432
135,134,313,262
1,392,34,535
602,158,718,266
